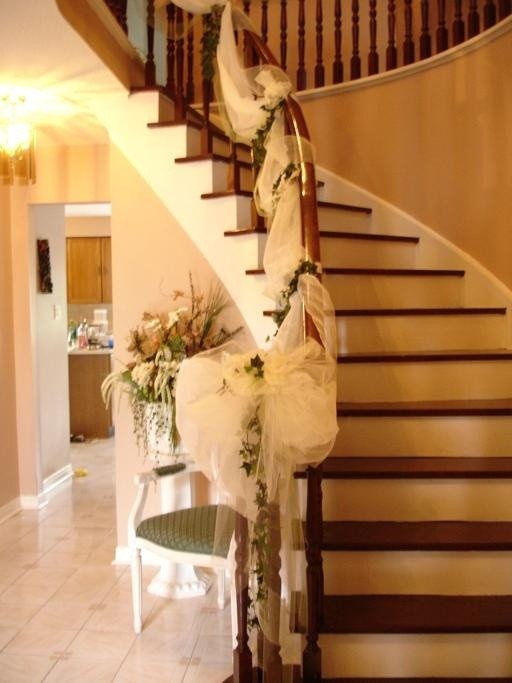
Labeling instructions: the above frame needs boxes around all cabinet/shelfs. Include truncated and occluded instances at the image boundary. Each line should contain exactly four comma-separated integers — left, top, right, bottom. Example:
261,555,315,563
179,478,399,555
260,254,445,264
68,355,115,439
65,236,111,304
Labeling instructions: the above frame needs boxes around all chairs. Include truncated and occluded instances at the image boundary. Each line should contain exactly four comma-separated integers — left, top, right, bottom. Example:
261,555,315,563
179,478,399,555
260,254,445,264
126,460,256,665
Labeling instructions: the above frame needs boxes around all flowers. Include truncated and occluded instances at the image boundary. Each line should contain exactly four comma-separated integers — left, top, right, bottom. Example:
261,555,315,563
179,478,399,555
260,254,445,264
98,268,246,418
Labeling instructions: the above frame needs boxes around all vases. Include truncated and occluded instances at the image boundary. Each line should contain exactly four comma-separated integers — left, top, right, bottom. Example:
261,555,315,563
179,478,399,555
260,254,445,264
131,397,182,455
